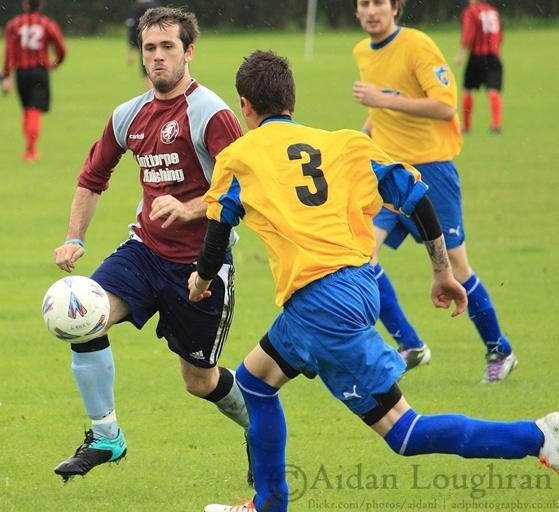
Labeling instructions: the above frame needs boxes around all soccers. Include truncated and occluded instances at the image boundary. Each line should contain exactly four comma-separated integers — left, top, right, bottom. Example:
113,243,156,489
42,274,110,345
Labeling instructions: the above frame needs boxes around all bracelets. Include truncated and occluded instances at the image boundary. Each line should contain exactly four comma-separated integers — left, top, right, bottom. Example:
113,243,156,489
194,274,207,290
63,239,85,248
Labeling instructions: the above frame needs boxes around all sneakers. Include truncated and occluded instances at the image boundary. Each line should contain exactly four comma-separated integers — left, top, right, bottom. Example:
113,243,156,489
480,350,519,386
533,410,559,479
203,492,263,512
396,342,432,377
53,426,130,484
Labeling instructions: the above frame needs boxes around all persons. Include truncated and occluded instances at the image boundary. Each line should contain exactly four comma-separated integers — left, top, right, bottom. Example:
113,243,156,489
350,0,519,382
0,0,69,160
453,0,504,135
186,49,559,512
52,9,248,473
